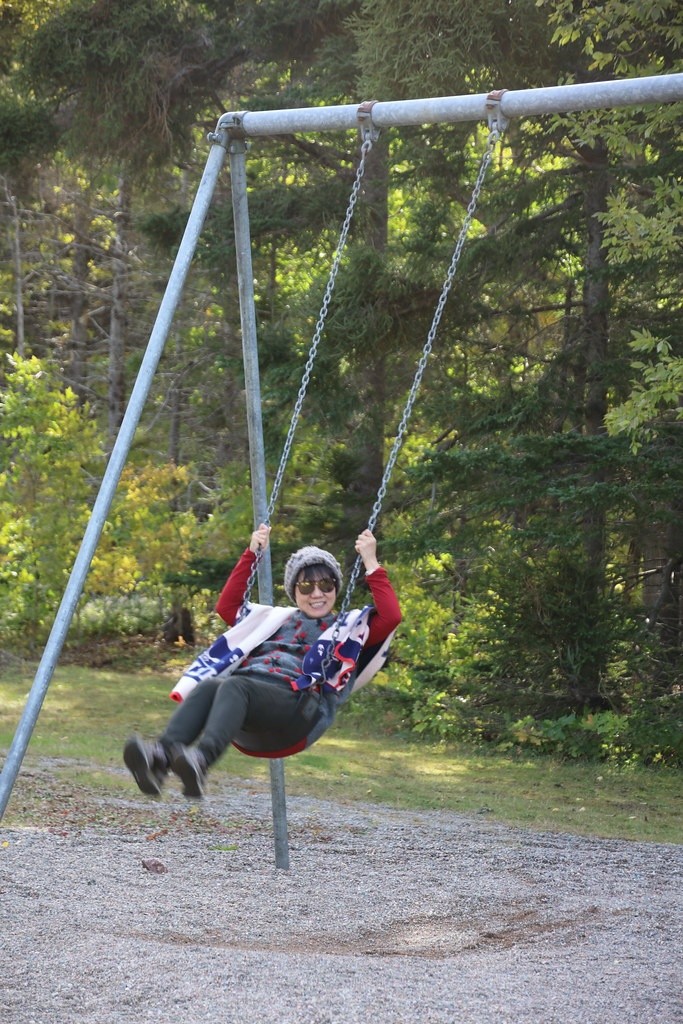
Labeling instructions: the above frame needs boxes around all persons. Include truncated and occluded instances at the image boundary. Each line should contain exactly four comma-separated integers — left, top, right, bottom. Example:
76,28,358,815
123,523,401,797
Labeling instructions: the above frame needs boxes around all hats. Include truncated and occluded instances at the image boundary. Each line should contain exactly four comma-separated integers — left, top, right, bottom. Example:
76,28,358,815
284,545,342,605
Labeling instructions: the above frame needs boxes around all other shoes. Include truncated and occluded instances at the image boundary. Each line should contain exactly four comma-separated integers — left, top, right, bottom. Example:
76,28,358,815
123,738,161,796
171,753,204,799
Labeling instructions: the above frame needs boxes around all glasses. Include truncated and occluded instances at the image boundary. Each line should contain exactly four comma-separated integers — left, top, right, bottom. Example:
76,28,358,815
294,578,336,595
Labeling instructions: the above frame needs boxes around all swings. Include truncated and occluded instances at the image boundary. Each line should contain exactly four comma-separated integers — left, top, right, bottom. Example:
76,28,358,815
226,119,503,753
